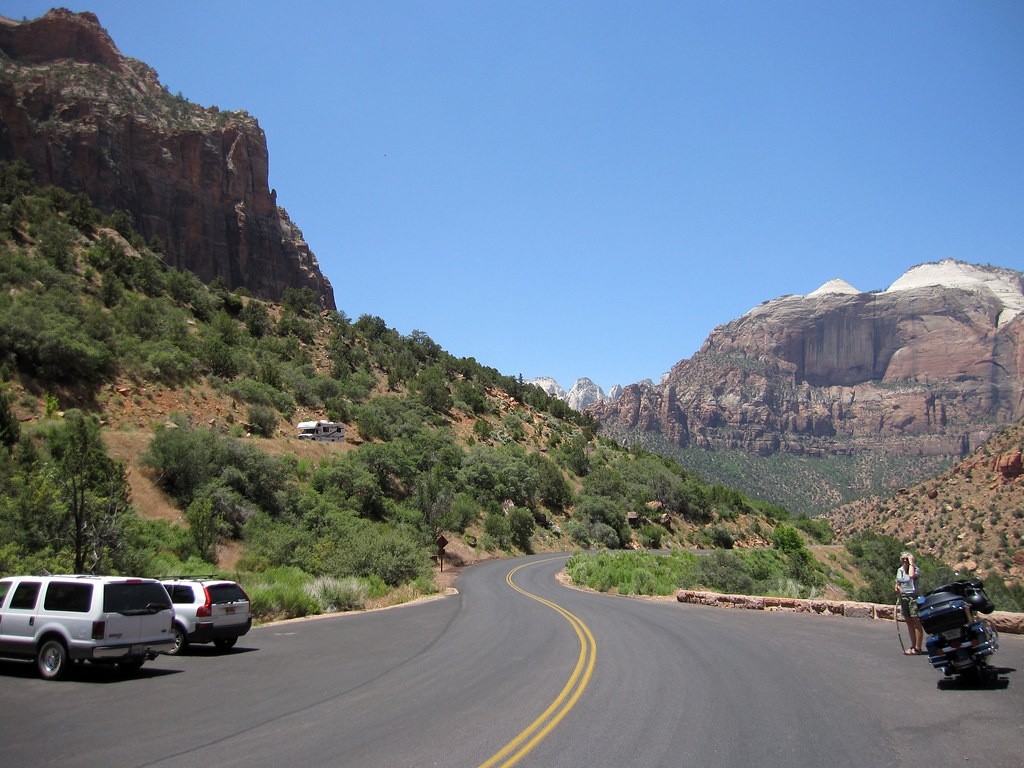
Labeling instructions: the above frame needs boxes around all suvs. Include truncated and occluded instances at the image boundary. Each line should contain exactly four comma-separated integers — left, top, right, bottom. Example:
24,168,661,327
139,574,252,655
0,575,176,680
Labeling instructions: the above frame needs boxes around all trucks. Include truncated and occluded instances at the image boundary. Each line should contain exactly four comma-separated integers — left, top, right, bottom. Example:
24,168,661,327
297,420,345,442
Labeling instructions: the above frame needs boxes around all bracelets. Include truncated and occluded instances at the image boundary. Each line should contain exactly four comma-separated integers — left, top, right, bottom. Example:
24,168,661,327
909,564,916,567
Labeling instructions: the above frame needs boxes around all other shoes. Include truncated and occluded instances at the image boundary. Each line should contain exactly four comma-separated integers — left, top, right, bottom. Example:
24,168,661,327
912,647,923,655
903,649,914,655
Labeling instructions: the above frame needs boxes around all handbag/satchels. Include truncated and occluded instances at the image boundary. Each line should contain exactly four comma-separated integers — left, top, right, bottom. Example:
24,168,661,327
916,595,925,604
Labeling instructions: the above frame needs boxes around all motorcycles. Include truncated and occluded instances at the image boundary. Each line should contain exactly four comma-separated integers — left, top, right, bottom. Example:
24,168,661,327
915,578,999,686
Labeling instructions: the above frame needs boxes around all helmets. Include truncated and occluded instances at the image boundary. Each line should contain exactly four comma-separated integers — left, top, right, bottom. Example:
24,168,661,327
981,600,995,614
969,593,986,611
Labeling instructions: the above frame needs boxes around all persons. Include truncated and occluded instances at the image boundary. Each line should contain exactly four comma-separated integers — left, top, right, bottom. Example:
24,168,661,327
895,552,924,656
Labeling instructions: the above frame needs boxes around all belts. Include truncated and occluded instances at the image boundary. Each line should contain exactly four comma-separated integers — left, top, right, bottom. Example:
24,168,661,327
900,593,914,597
